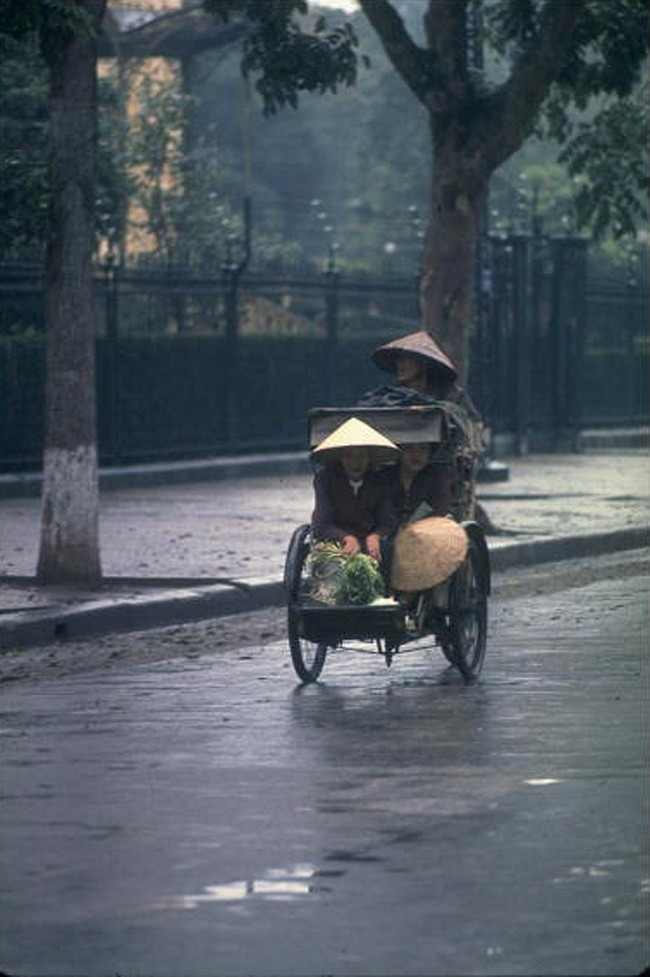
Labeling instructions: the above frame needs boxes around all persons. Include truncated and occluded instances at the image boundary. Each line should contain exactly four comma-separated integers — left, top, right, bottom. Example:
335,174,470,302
312,417,452,611
373,330,481,519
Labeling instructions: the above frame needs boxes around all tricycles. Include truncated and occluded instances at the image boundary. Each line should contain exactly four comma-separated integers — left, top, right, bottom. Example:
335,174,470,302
284,382,500,684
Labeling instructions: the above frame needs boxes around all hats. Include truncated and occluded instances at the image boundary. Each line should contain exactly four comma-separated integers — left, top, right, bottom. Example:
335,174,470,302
314,417,399,455
390,516,468,592
371,331,457,381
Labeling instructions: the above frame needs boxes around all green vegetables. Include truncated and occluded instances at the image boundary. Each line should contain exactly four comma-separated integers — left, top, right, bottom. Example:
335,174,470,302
314,541,380,605
